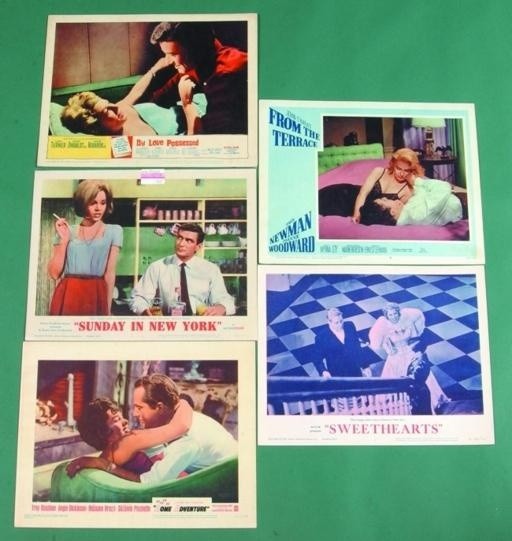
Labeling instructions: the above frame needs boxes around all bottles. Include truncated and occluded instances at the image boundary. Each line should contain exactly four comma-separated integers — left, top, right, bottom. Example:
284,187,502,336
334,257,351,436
149,297,164,315
168,287,186,316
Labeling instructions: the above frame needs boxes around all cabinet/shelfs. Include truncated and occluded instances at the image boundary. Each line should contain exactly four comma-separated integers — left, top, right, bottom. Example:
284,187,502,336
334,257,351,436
134,198,247,316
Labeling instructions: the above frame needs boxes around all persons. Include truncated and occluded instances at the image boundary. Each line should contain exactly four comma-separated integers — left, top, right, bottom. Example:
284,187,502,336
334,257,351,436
312,308,372,378
47,179,124,316
65,373,238,484
60,55,200,136
76,398,194,481
368,303,452,415
318,147,425,225
358,172,468,226
127,222,237,317
146,22,248,135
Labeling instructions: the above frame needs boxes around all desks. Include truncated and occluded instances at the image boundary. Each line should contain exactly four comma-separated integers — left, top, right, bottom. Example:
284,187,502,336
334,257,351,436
171,379,238,427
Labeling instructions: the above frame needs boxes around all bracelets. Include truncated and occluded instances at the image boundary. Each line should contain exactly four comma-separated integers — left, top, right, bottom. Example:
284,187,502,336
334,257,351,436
107,462,116,473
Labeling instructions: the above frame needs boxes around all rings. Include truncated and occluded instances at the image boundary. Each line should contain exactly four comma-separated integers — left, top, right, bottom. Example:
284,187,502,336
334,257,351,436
76,464,80,470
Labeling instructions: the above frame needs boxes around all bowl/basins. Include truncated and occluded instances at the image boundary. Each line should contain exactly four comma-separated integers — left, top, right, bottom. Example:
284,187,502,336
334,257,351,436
222,240,238,247
205,241,220,247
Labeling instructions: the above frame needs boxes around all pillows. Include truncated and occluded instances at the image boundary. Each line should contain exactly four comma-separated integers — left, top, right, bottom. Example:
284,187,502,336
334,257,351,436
48,102,93,136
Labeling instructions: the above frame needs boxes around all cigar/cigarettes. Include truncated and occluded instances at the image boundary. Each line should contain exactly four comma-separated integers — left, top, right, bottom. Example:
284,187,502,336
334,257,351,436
52,212,60,220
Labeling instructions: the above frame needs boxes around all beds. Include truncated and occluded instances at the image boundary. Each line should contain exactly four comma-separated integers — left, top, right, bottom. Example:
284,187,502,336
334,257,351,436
417,154,457,179
318,158,469,240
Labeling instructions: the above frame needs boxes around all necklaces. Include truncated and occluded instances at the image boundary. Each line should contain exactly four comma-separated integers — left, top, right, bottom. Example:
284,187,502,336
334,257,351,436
82,224,102,246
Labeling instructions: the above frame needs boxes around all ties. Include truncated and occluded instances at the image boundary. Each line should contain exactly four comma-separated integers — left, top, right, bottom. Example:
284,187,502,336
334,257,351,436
179,262,194,315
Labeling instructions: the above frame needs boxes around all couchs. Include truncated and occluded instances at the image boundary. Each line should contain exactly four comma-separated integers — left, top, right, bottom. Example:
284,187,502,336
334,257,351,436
50,457,238,502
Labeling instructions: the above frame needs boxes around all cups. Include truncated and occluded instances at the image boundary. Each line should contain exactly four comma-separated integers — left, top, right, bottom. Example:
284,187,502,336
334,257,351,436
195,302,209,316
232,207,239,218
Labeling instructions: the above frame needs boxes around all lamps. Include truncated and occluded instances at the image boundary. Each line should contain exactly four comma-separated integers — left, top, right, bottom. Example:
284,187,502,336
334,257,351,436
411,118,447,156
